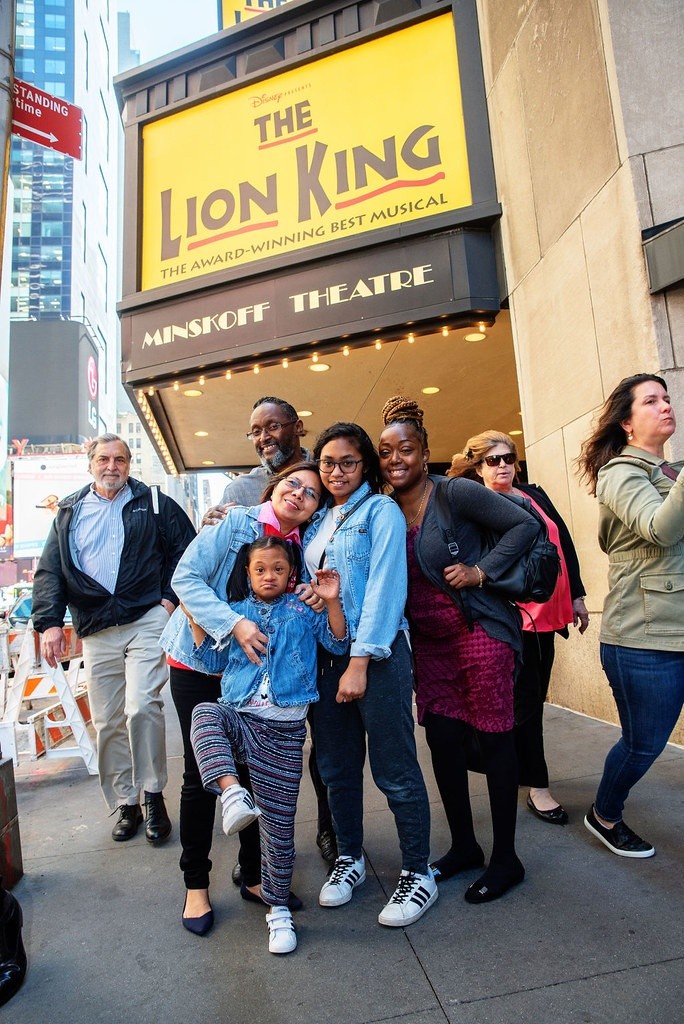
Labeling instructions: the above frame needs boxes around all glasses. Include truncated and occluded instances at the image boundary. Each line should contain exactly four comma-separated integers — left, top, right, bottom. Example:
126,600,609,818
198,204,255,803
478,452,517,467
316,458,363,474
246,420,297,441
282,476,321,502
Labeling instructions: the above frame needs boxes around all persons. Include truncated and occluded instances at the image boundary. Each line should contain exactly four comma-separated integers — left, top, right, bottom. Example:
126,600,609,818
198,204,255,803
445,429,590,826
568,372,684,859
0,839,28,1009
30,432,197,848
157,460,332,938
179,534,351,955
298,421,441,929
377,393,542,905
200,395,338,887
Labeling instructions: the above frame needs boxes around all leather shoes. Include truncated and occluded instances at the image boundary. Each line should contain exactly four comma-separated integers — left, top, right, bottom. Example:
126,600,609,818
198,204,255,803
316,828,337,860
232,863,243,884
525,790,570,825
141,791,172,843
108,804,144,841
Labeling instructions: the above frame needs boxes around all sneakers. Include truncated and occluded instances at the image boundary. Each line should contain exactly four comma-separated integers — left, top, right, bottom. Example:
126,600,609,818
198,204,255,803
378,865,439,926
584,802,655,858
319,854,366,907
265,906,297,953
221,783,262,836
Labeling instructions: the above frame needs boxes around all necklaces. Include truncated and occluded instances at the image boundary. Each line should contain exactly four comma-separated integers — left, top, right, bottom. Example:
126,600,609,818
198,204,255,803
390,480,428,525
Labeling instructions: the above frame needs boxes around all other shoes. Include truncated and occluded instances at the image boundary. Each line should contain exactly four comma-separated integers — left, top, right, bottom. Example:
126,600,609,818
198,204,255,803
428,845,485,883
181,890,215,936
240,882,305,911
464,858,525,903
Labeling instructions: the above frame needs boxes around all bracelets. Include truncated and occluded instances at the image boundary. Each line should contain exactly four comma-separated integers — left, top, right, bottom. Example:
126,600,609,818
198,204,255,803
577,596,586,601
474,564,483,589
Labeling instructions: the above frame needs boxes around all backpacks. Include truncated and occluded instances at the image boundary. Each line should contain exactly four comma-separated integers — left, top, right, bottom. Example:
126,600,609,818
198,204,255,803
434,476,563,604
